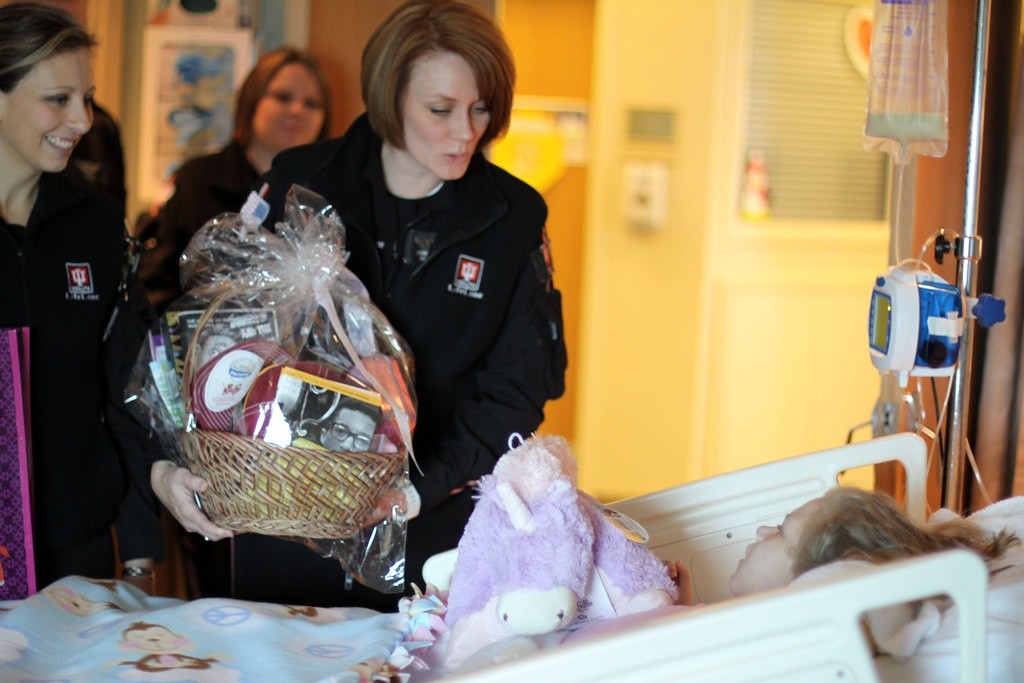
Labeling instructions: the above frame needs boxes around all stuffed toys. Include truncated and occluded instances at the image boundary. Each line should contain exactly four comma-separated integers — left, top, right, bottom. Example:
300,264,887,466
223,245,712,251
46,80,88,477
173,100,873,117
433,427,681,670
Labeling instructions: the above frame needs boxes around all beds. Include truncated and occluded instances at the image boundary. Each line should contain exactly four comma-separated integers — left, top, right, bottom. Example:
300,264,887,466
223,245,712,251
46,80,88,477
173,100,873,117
0,432,1023,683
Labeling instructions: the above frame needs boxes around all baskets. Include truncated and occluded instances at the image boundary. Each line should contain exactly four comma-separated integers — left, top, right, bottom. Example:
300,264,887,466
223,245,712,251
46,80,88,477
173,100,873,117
179,284,418,539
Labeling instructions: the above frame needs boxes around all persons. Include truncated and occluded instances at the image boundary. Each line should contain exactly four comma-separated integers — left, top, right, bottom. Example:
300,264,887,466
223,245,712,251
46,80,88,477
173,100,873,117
135,44,330,316
415,483,1020,677
319,399,381,455
133,0,568,616
0,4,179,601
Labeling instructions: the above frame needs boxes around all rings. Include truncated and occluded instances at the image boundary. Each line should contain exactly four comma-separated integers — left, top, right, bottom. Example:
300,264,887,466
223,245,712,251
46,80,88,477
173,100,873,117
205,537,209,542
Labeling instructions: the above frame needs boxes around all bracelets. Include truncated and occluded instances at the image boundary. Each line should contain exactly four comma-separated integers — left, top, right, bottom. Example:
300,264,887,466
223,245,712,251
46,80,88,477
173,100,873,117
122,566,152,577
